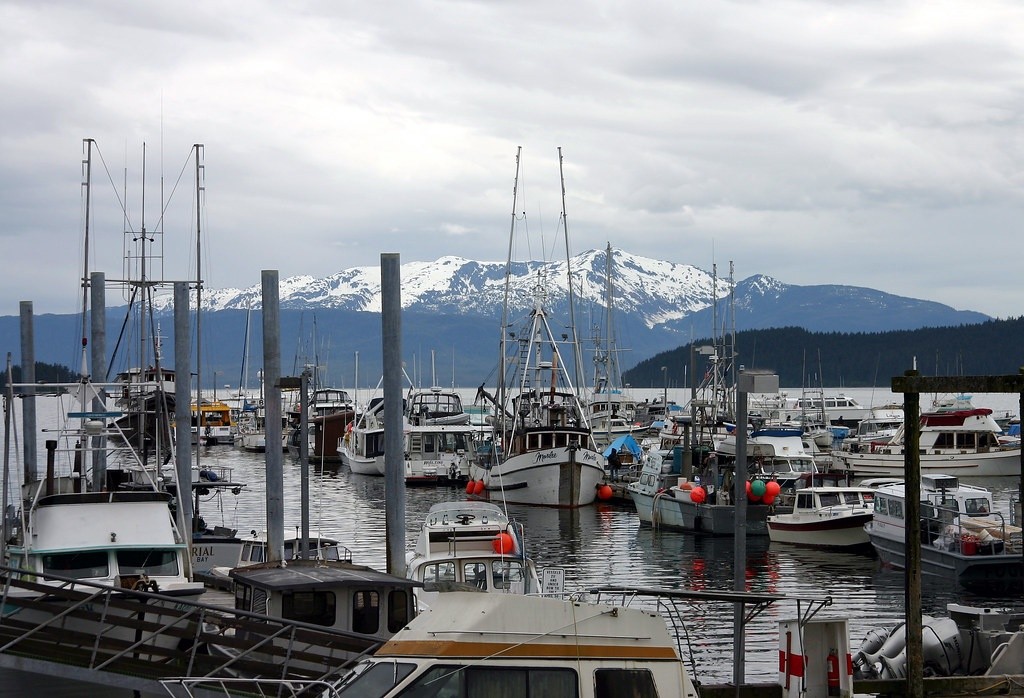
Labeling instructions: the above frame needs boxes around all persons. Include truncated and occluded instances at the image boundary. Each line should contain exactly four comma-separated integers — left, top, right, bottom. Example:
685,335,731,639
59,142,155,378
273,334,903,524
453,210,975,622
607,448,620,483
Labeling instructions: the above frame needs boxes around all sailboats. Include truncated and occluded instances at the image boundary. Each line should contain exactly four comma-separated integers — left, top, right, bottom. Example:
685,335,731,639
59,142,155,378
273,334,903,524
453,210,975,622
0,134,1023,698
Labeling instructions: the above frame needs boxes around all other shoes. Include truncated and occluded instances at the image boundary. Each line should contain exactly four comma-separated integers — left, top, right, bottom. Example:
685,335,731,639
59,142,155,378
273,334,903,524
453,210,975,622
610,480,614,483
614,479,619,483
